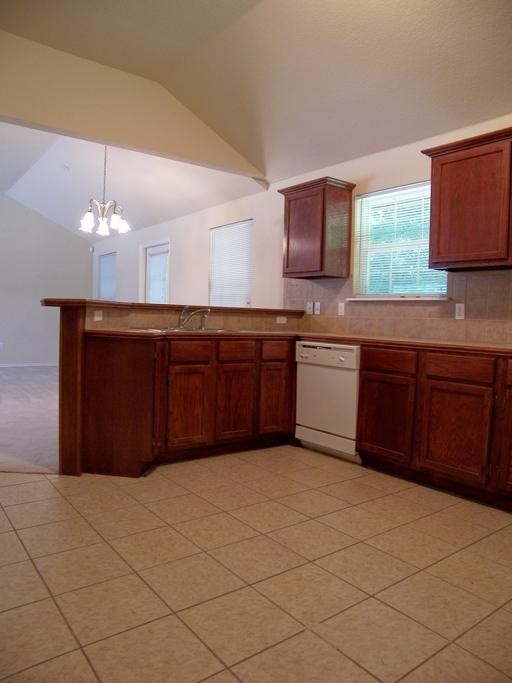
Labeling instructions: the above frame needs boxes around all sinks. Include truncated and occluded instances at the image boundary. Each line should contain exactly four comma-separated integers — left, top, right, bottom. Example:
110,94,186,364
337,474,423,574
149,329,224,332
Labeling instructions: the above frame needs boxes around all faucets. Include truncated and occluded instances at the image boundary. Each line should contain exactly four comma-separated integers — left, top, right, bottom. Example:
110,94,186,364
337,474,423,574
179,305,211,329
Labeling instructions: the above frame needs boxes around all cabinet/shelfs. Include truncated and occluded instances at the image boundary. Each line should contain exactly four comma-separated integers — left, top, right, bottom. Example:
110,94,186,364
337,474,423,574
357,330,512,512
278,176,356,280
84,328,296,478
420,126,512,273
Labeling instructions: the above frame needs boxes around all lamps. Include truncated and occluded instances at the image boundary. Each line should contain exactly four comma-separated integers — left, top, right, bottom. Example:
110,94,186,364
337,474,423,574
78,145,131,237
